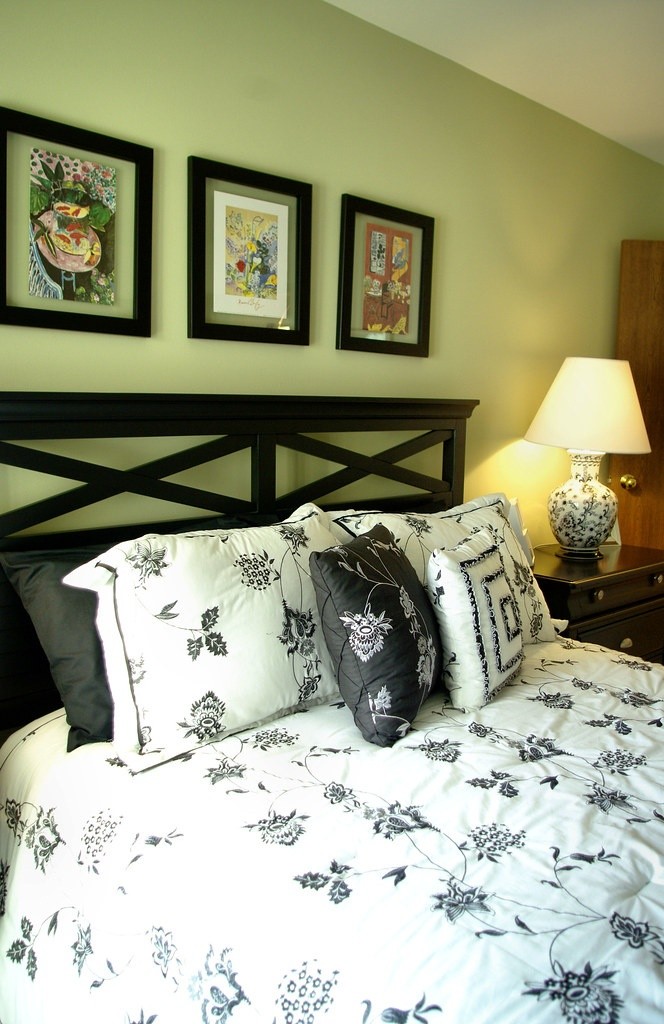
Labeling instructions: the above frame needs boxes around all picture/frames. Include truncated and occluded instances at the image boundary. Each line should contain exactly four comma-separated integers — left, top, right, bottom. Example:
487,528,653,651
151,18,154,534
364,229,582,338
335,194,435,357
187,155,313,346
0,107,154,337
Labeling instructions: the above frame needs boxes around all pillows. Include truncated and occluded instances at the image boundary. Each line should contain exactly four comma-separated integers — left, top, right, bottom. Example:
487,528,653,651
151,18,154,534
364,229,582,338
61,502,344,776
324,493,569,645
426,523,527,712
0,542,112,753
308,522,444,747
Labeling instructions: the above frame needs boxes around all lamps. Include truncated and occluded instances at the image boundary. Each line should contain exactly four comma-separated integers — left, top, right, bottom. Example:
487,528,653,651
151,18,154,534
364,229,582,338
524,357,652,561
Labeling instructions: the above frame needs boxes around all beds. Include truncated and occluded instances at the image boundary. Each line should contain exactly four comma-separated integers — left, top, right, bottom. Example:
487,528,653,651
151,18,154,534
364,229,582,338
0,391,664,1024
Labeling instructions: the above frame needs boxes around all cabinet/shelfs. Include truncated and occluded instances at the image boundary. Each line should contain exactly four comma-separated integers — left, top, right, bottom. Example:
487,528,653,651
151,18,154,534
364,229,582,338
530,543,664,666
